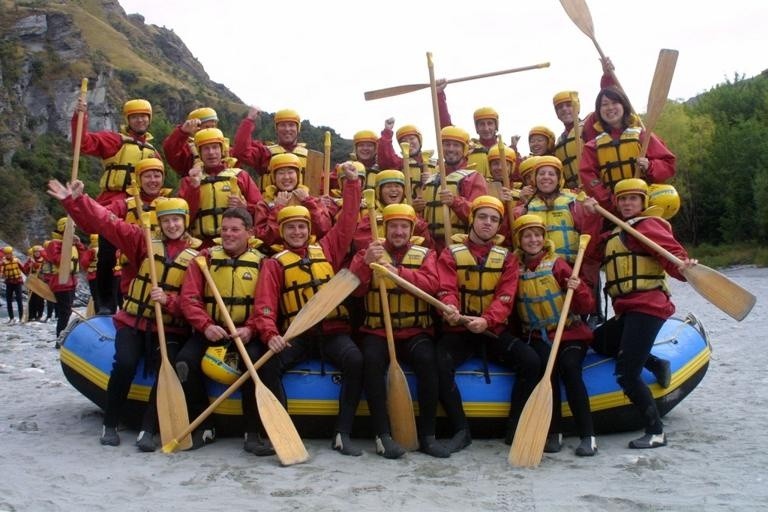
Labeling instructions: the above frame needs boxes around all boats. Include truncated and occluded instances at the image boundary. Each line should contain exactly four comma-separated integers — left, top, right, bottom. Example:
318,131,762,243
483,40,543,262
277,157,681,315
60,312,712,440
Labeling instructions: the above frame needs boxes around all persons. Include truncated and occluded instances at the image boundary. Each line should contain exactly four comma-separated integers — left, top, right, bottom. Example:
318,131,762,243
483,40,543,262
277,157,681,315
0,56,700,460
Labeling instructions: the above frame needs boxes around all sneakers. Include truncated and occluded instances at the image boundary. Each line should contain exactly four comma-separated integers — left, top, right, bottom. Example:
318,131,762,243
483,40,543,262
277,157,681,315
652,359,670,388
189,427,215,450
542,433,563,453
100,424,119,446
331,432,475,458
244,432,275,456
575,436,596,456
136,431,155,452
586,314,605,330
628,432,666,449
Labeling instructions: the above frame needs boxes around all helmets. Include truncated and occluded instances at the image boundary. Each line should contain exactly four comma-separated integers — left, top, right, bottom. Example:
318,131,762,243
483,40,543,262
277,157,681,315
200,346,242,386
648,183,680,220
613,178,648,208
552,91,579,113
122,99,311,238
337,107,562,248
3,217,98,254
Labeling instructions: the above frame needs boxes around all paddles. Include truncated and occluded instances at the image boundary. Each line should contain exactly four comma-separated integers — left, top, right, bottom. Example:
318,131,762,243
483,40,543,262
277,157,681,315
364,189,420,452
365,62,549,101
305,149,323,195
634,50,678,178
507,234,592,468
58,77,88,285
578,190,757,321
371,262,499,343
561,0,633,112
25,273,86,318
142,212,193,452
194,255,310,466
160,266,361,454
